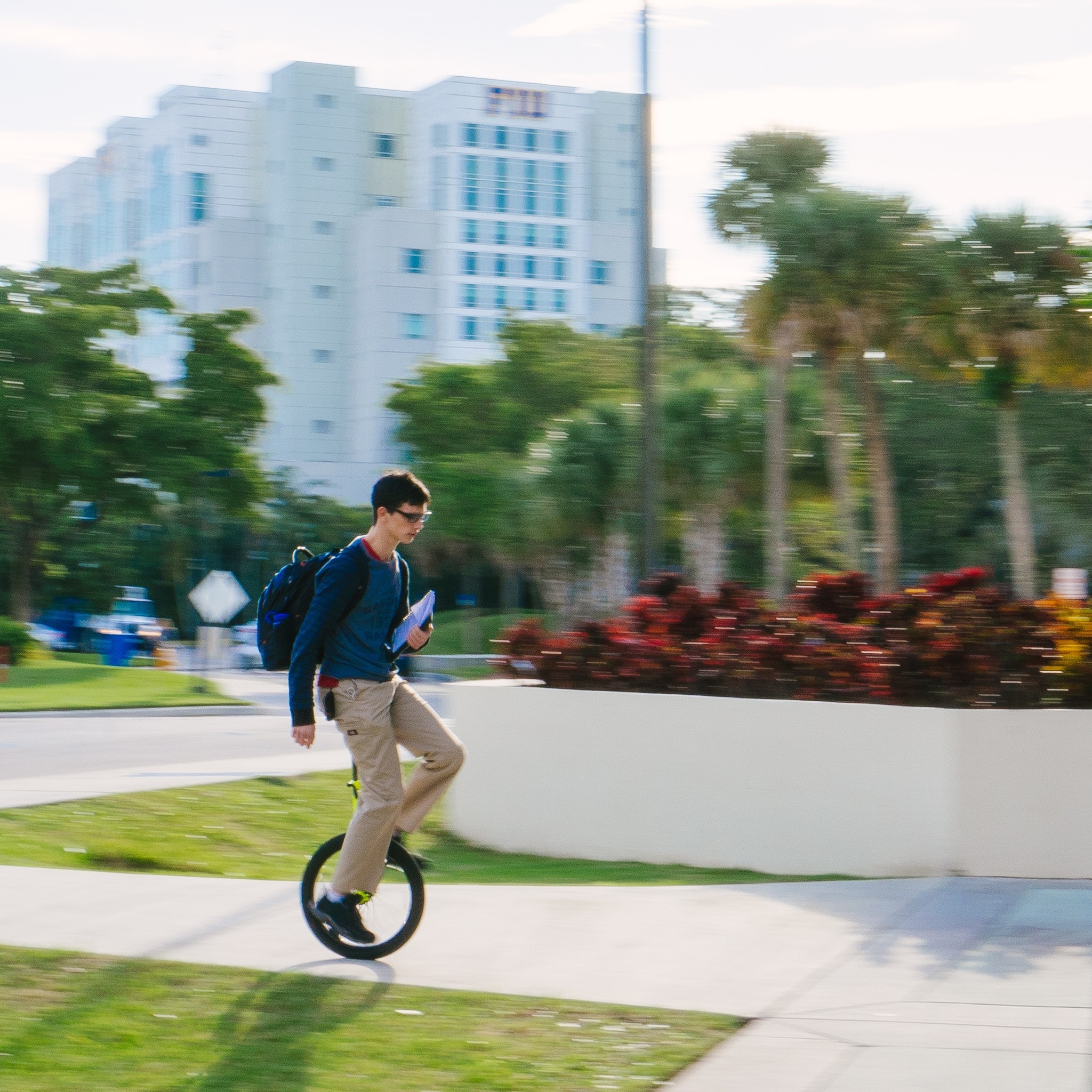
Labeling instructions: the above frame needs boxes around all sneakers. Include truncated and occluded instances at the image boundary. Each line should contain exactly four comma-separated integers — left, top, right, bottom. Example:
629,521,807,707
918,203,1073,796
383,840,431,875
311,895,374,945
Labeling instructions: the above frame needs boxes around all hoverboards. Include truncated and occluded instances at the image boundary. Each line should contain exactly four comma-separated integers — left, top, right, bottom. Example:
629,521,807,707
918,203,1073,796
297,754,428,960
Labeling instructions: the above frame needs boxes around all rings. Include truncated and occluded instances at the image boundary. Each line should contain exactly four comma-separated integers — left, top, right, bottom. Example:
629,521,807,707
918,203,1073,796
304,738,309,742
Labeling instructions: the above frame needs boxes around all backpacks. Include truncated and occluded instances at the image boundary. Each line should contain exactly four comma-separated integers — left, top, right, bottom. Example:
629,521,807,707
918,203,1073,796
257,543,410,673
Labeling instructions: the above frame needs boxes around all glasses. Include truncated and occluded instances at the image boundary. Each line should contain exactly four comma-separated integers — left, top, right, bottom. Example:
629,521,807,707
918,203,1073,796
386,505,433,524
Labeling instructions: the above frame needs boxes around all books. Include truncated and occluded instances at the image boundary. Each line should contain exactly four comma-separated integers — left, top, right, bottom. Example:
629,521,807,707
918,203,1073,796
389,587,435,662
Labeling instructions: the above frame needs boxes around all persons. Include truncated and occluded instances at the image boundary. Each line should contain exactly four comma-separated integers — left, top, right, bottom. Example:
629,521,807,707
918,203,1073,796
288,470,462,946
